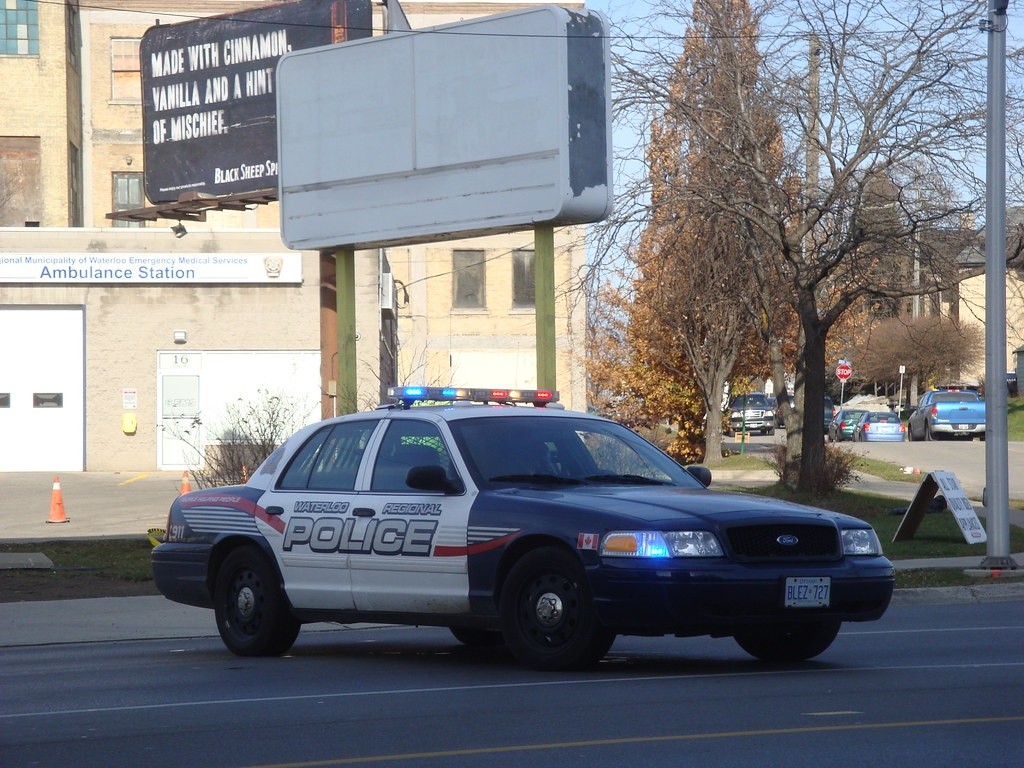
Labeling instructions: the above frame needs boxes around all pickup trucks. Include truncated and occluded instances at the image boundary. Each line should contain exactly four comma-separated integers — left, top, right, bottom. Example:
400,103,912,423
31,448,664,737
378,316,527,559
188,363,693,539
907,389,986,441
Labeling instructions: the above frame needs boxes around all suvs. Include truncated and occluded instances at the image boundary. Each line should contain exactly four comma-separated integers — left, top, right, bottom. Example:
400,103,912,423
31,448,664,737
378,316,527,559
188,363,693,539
824,397,836,430
723,392,775,437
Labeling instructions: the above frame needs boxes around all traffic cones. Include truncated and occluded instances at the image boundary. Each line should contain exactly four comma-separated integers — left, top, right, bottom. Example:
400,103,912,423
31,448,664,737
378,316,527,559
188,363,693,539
178,472,191,495
240,466,248,484
47,476,71,523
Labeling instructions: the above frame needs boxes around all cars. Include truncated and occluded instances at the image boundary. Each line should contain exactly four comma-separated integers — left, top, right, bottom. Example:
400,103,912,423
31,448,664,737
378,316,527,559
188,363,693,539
892,403,905,413
828,408,868,442
853,411,905,442
771,395,795,429
150,384,896,670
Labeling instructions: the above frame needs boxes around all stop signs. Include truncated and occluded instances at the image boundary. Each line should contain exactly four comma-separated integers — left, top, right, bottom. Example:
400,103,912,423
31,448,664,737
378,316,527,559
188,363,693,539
836,365,852,380
